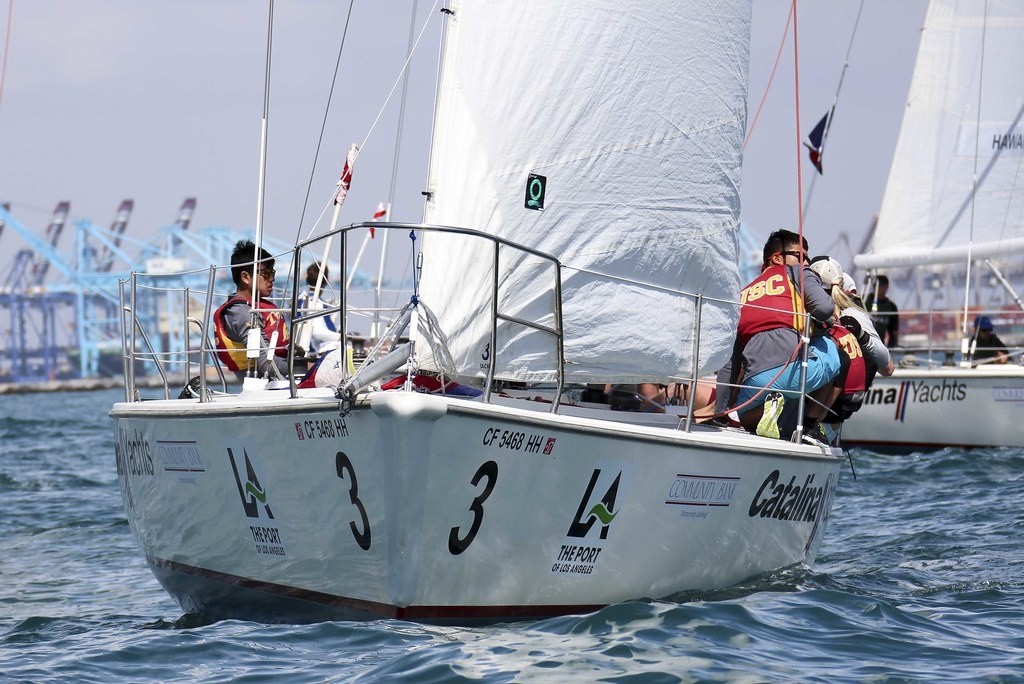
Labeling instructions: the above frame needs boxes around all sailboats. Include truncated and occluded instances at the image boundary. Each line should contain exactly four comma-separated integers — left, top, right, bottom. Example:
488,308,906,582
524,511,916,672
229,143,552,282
708,1,1024,458
105,1,848,627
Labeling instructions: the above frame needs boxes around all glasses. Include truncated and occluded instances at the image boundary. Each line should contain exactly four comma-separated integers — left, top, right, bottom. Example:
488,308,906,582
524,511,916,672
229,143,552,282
780,251,811,263
982,328,993,333
258,269,275,279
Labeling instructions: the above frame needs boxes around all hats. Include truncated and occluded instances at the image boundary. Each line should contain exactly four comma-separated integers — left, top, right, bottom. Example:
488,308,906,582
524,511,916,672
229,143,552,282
842,273,856,294
975,316,995,329
809,256,842,289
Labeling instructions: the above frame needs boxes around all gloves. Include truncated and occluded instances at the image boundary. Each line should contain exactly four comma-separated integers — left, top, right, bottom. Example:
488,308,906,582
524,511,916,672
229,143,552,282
841,315,864,339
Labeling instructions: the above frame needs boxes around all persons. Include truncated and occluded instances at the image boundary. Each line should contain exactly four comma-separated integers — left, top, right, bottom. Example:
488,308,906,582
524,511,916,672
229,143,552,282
711,229,895,445
579,377,716,410
213,241,315,376
969,316,1013,365
862,275,899,347
289,263,341,351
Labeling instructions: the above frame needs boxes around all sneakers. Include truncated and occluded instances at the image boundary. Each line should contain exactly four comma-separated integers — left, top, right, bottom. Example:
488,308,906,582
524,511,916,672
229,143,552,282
756,393,785,439
803,420,829,447
